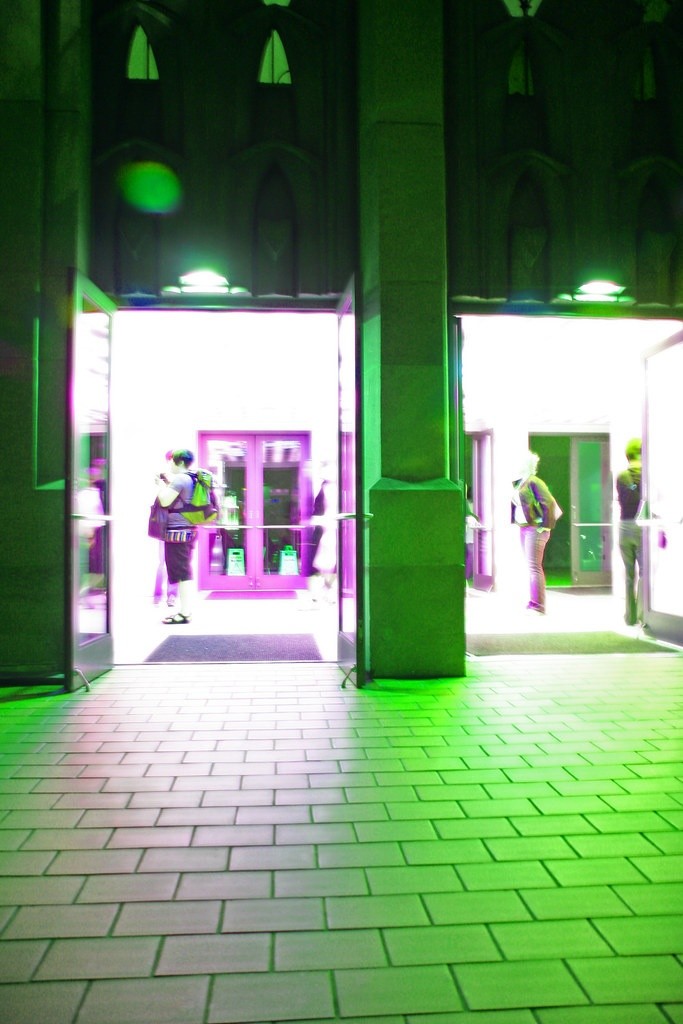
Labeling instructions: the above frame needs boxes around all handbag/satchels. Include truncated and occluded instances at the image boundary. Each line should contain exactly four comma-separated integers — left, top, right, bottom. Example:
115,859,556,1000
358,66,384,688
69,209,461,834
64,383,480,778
147,496,170,542
528,475,563,521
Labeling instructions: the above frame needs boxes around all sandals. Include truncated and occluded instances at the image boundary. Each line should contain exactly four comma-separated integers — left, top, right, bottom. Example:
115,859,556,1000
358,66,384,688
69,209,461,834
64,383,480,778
162,611,193,625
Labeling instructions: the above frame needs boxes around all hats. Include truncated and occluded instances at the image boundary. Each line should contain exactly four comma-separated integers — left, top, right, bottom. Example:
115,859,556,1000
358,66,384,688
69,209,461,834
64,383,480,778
165,448,194,462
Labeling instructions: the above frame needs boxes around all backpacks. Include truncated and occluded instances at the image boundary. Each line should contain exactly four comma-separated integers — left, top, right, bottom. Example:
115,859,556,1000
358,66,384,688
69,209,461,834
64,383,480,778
168,466,220,525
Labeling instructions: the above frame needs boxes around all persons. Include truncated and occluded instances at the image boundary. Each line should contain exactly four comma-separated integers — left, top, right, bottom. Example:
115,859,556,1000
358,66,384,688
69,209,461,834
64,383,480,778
508,449,556,617
154,449,199,625
310,480,339,587
77,459,108,610
465,486,486,596
616,438,652,626
